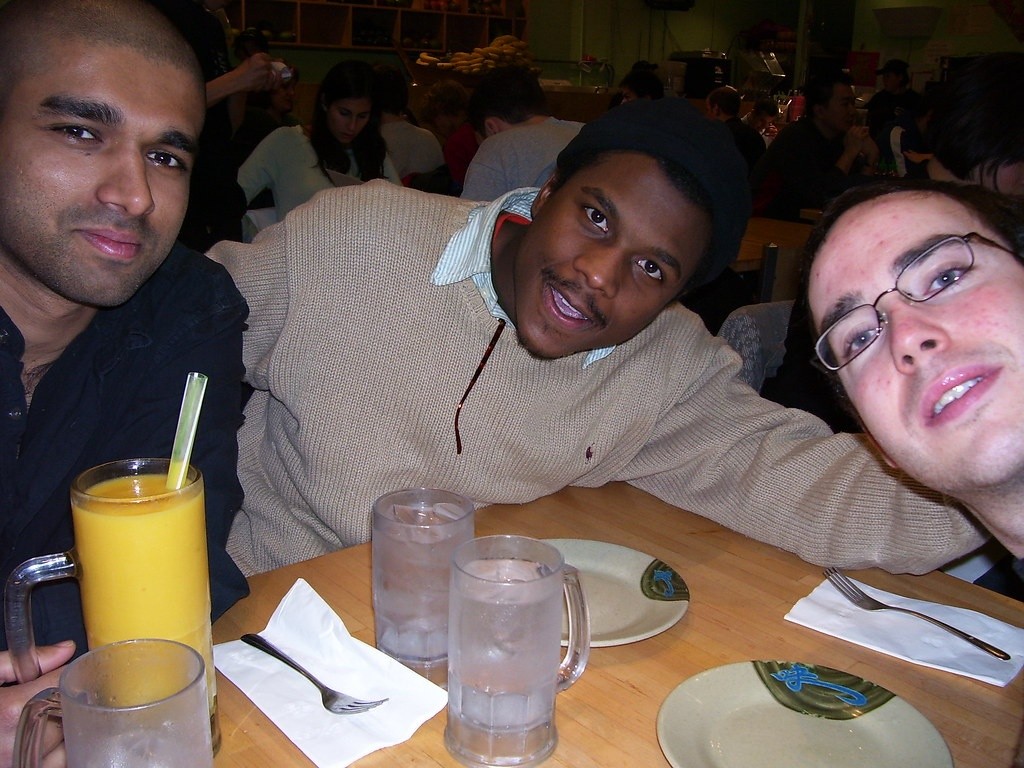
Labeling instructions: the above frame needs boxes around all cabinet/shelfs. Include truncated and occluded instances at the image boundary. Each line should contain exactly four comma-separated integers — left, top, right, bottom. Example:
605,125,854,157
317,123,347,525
223,0,530,54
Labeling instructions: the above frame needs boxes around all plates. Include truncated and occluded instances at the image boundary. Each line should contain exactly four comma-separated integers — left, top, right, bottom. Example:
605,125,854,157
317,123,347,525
538,538,689,647
655,658,954,767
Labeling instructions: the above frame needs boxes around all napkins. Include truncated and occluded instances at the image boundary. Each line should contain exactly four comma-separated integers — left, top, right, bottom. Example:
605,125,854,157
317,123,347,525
213,577,449,768
783,572,1024,687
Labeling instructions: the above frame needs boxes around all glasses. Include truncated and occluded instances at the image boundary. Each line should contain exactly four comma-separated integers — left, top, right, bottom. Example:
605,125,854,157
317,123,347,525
812,231,1024,372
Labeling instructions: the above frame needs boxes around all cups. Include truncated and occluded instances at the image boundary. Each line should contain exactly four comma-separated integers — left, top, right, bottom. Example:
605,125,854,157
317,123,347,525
11,638,213,768
4,457,223,759
444,531,589,767
369,490,474,666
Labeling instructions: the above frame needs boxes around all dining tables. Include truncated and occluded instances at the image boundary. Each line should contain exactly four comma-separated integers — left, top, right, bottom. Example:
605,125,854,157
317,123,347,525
213,480,1024,768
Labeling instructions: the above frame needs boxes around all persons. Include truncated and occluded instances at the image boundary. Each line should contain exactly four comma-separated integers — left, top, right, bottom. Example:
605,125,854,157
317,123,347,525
147,0,593,252
202,109,993,578
0,0,251,768
794,181,1024,601
610,51,1024,223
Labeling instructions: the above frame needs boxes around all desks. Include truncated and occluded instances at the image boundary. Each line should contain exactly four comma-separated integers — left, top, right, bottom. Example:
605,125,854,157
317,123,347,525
719,216,817,274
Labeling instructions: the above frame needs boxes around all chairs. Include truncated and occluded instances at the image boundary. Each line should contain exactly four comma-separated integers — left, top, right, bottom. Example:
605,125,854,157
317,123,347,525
682,237,777,398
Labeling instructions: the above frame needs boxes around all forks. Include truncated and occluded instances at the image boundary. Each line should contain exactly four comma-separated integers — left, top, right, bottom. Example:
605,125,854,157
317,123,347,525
823,566,1012,661
241,633,389,714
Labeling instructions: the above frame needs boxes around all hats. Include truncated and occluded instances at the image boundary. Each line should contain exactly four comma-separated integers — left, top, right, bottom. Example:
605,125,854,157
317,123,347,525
875,59,909,74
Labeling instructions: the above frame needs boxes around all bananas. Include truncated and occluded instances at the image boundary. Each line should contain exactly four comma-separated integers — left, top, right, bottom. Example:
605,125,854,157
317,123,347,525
415,35,533,73
